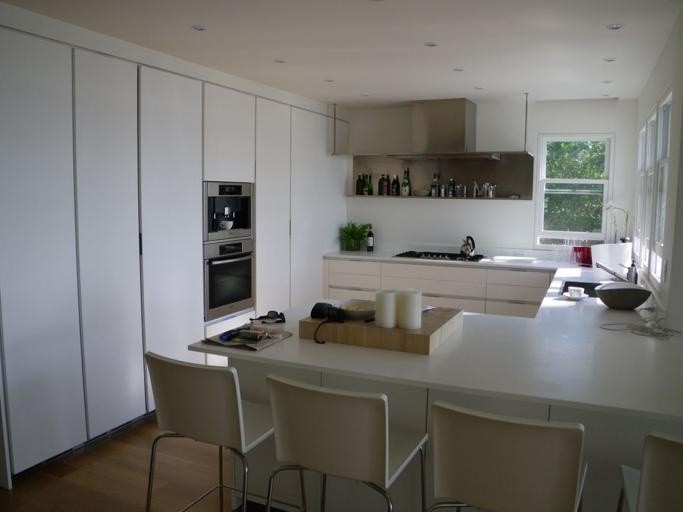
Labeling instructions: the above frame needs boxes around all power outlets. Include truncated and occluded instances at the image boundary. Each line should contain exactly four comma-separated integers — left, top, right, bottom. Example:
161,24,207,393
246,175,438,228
648,294,657,319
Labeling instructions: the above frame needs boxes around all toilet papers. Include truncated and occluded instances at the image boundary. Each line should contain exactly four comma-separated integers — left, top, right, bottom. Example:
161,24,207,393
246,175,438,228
398,288,422,329
375,290,398,329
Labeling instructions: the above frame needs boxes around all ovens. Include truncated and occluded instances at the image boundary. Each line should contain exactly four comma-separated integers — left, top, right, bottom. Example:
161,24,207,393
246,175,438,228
201,180,255,323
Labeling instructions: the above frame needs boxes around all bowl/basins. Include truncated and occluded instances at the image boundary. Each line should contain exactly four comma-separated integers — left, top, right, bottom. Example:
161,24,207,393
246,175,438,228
332,299,375,319
594,284,652,309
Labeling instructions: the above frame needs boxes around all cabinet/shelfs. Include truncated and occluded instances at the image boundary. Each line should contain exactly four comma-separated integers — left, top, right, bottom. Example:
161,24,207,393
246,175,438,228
204,81,255,184
137,59,203,415
322,256,378,300
486,270,551,318
378,264,486,316
254,96,352,320
0,28,149,484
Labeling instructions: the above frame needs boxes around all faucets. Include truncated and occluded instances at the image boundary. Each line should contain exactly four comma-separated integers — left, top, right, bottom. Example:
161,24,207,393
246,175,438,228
595,261,628,282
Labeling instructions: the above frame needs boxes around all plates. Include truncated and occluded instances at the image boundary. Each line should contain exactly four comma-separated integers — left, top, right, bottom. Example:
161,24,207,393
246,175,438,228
562,292,589,300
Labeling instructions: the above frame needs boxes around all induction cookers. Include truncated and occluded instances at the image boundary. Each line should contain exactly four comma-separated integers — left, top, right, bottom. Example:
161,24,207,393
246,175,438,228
395,250,483,262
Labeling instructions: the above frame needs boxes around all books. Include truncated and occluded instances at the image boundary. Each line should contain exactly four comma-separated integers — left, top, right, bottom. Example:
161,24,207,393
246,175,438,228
201,324,293,351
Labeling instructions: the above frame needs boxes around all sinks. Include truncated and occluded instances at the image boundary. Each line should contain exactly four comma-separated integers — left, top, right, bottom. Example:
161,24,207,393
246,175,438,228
559,279,602,300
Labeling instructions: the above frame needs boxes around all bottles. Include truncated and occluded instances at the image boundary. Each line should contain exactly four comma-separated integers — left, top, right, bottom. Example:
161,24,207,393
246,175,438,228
428,174,455,198
366,224,374,251
627,262,636,284
354,168,410,196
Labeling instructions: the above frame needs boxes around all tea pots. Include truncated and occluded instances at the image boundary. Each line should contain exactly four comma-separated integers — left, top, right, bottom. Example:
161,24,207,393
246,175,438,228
458,235,476,258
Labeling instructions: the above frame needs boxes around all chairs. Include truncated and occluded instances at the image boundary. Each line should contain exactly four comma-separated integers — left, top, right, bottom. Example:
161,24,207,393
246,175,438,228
613,432,682,512
130,350,304,511
421,399,591,510
260,369,429,511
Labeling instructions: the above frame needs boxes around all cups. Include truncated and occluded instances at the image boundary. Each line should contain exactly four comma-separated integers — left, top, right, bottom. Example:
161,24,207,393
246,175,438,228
567,286,584,297
487,186,494,198
218,220,233,230
373,289,421,330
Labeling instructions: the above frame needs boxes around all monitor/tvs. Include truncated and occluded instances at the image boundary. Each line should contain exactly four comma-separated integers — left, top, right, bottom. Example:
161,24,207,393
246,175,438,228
204,253,256,322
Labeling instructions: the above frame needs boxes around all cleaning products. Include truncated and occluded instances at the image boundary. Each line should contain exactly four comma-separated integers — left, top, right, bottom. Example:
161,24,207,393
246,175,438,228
448,179,454,197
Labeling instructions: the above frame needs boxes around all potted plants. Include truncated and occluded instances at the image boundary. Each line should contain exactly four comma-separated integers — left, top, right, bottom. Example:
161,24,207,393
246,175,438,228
337,222,369,252
